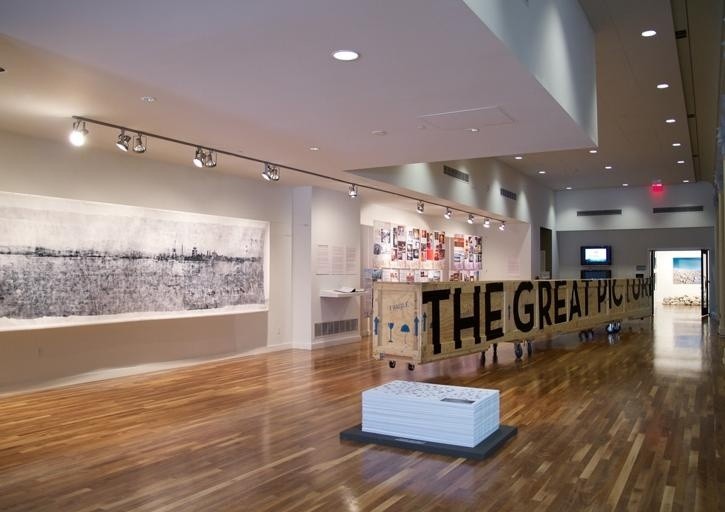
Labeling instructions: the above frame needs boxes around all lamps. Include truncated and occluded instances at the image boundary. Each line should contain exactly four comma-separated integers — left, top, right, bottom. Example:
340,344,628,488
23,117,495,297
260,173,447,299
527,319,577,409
68,115,507,233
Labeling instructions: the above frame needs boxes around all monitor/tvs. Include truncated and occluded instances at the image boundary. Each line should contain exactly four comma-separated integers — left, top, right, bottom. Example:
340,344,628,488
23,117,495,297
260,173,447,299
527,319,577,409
580,245,613,266
580,269,611,279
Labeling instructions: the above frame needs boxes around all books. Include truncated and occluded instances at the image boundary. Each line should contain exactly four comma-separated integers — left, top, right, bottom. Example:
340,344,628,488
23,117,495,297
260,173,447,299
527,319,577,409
334,285,365,293
